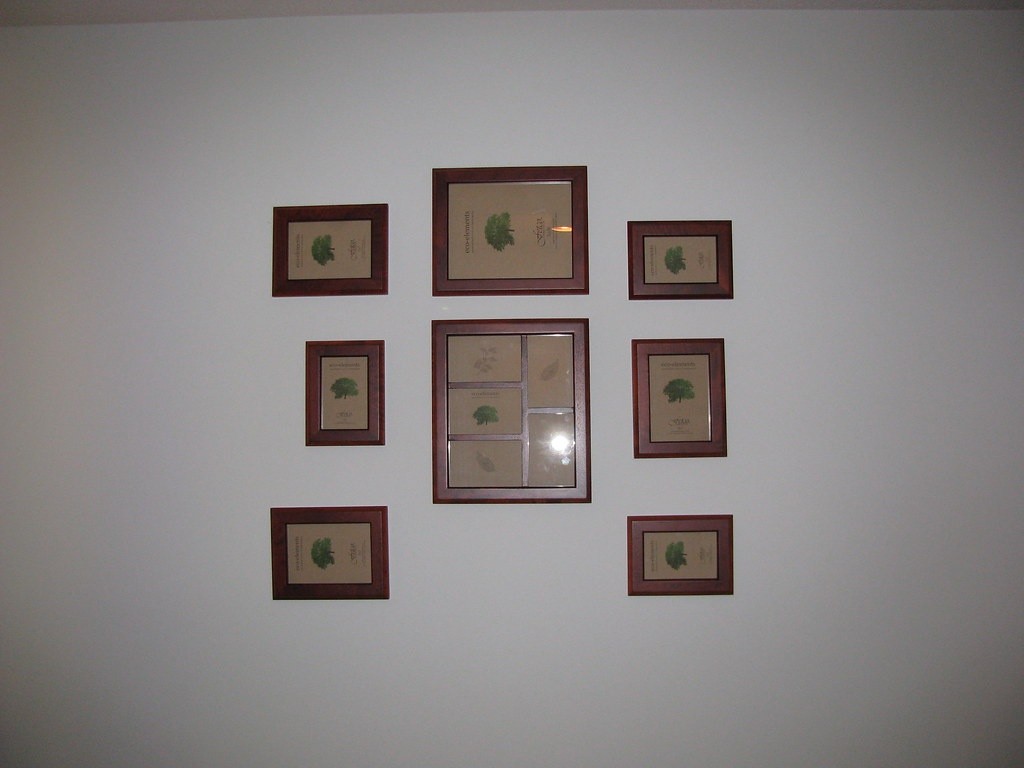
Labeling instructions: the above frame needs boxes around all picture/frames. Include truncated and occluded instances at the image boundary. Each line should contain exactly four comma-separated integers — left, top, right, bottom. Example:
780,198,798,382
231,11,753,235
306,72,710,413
627,220,733,299
631,338,727,457
627,515,733,596
431,318,592,504
432,165,589,295
305,340,385,446
271,506,390,600
272,202,387,296
